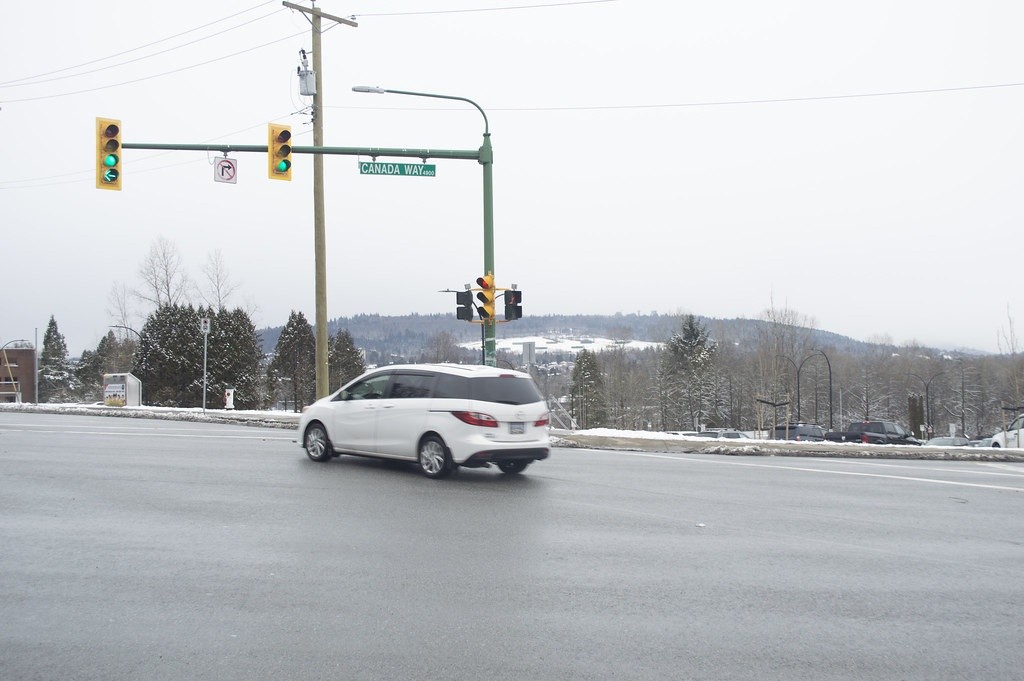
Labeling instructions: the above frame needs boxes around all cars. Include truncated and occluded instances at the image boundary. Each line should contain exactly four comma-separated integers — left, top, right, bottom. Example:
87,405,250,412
918,414,1024,449
296,363,551,481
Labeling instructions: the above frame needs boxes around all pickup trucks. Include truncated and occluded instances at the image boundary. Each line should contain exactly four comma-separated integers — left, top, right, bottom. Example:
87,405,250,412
826,421,923,445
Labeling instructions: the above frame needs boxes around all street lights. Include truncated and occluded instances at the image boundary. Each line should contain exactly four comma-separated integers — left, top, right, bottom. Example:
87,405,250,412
807,348,832,431
353,86,496,371
907,372,948,440
775,354,823,420
109,325,147,406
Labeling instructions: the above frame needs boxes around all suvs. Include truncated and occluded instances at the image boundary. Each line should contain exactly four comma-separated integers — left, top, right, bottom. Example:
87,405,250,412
766,422,825,442
667,423,753,442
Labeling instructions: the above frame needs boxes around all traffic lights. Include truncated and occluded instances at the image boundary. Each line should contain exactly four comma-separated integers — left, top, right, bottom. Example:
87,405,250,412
505,290,523,320
476,276,495,319
268,123,294,181
456,290,473,320
95,114,124,190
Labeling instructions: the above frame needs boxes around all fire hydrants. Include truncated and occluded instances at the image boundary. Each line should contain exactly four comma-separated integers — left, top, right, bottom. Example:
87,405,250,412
225,383,235,409
